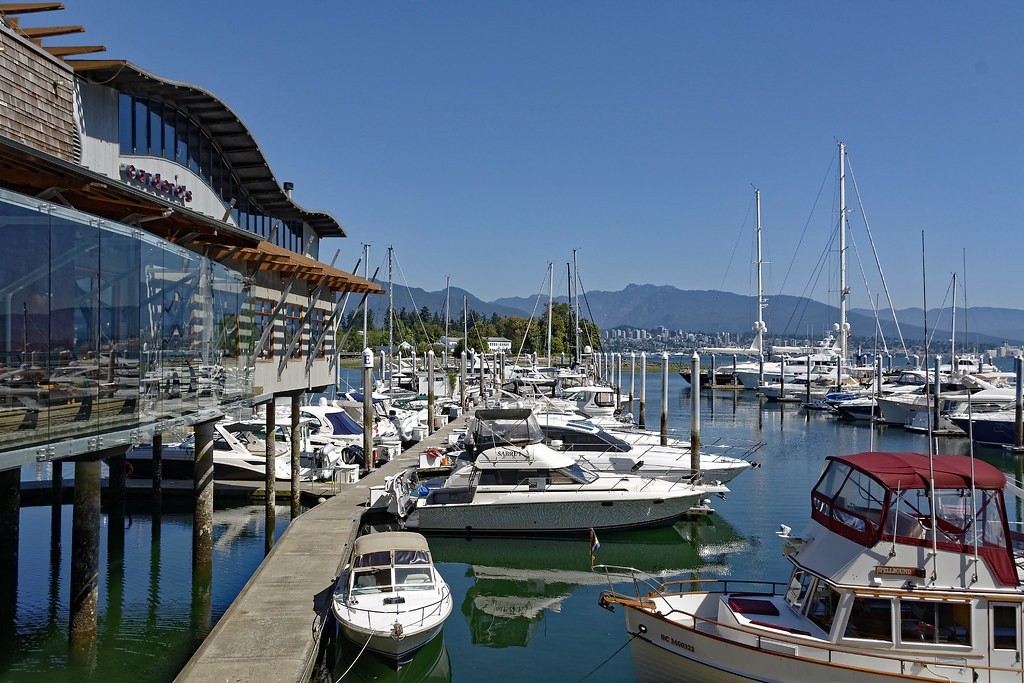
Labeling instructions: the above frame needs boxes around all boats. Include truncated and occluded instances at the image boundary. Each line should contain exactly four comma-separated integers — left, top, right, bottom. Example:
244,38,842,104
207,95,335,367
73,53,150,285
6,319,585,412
328,637,453,683
0,300,227,402
330,528,452,665
99,131,1023,533
594,227,1023,683
